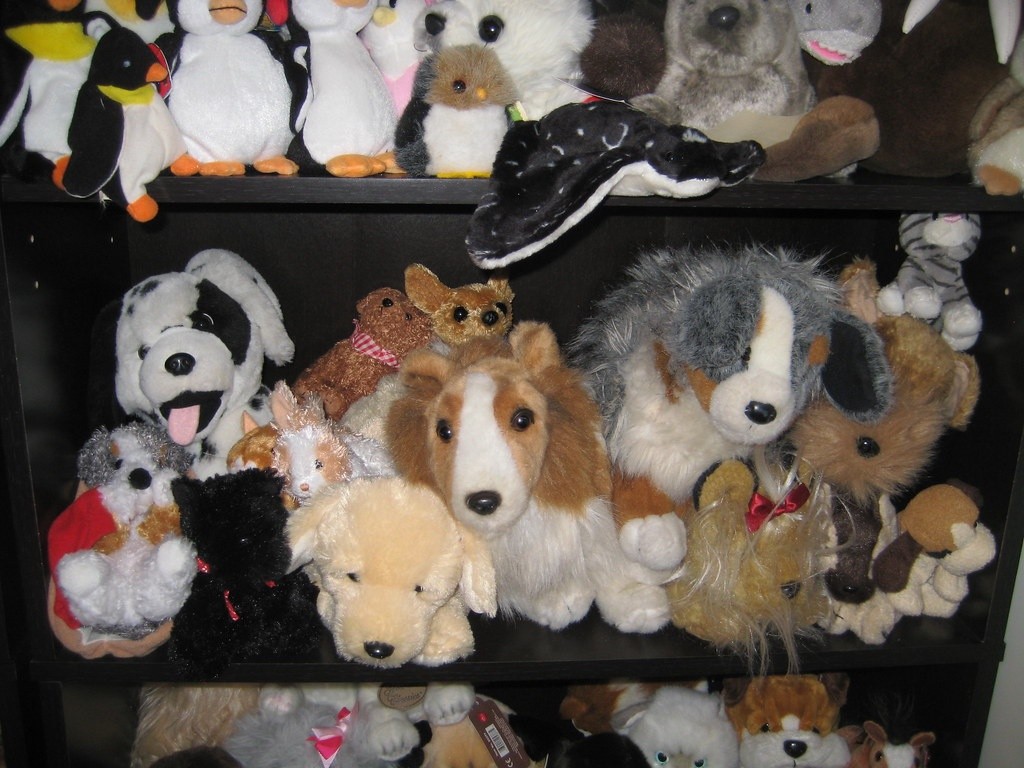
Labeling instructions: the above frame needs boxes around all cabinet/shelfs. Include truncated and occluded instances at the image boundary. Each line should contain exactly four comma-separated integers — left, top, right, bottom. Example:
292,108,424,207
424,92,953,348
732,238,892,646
0,0,1024,768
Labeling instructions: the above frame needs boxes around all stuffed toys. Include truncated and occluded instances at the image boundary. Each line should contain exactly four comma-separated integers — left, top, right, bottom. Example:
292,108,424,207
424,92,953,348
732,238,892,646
40,215,997,662
0,0,1024,269
129,671,939,768
